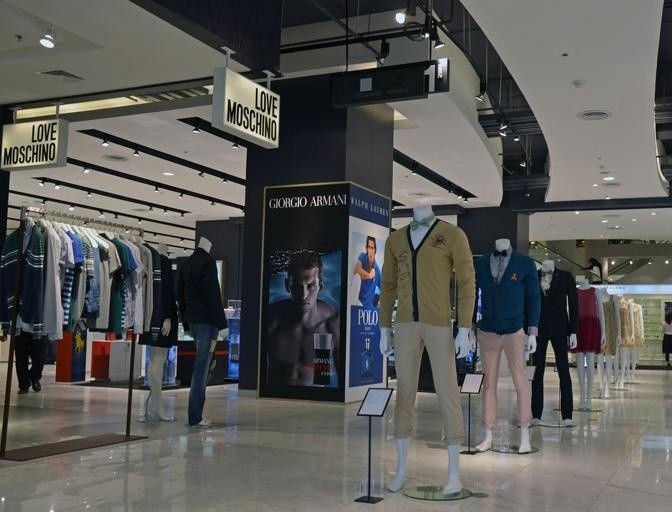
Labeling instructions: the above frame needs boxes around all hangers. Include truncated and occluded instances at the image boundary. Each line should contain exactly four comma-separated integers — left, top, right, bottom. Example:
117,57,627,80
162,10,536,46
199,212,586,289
26,208,143,238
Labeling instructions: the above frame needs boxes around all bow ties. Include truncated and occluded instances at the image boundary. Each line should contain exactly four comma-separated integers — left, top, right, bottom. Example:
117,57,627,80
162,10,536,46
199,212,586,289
541,270,553,276
493,249,508,256
409,216,435,231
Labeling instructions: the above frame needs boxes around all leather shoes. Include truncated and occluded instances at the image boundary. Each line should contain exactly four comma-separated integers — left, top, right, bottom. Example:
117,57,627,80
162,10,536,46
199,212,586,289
18,389,29,394
32,380,41,391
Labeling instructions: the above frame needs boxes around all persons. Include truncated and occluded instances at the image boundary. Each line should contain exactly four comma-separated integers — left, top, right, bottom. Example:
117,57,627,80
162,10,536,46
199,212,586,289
378,205,479,496
180,235,228,424
570,278,605,411
353,236,384,306
269,250,340,384
587,259,603,284
583,257,603,284
473,239,544,454
1,326,48,395
137,242,180,422
598,284,645,399
531,258,580,429
661,312,672,367
